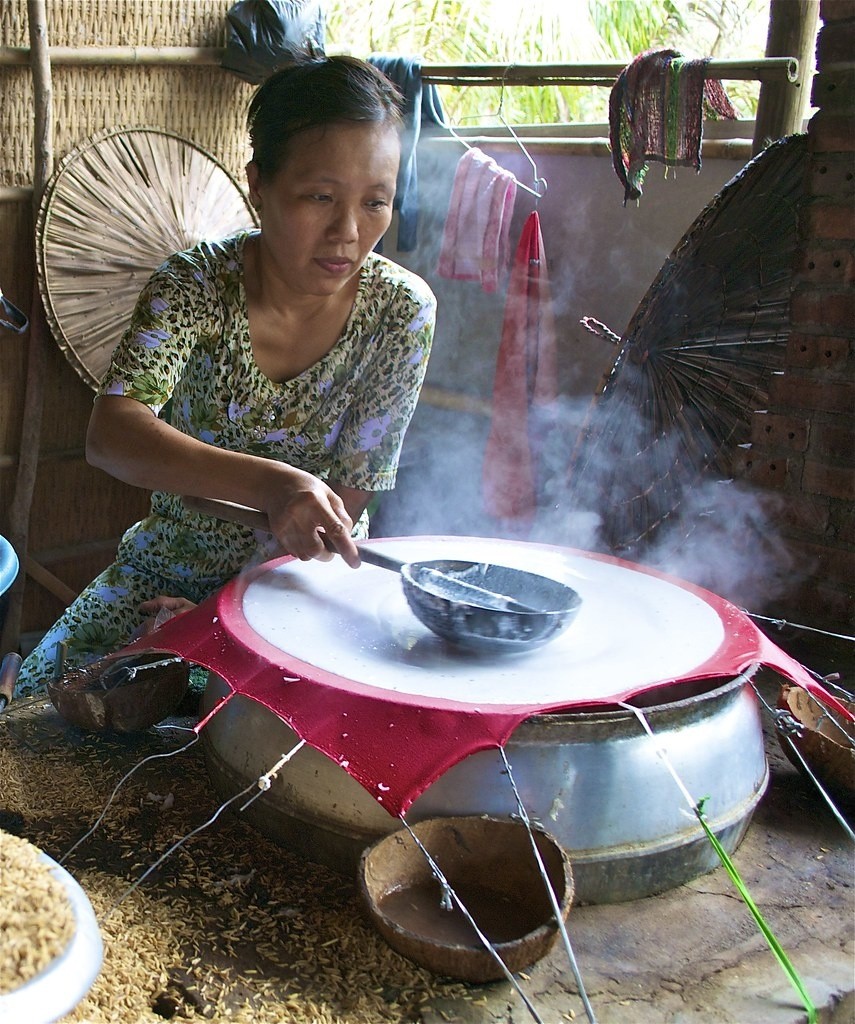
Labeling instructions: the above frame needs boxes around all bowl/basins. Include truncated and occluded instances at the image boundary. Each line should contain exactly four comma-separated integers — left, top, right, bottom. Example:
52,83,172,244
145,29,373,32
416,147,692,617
775,686,855,802
49,653,191,731
361,819,573,983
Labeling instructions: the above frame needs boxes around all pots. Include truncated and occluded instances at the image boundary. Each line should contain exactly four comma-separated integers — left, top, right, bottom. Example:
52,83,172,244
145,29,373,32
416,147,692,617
204,661,769,908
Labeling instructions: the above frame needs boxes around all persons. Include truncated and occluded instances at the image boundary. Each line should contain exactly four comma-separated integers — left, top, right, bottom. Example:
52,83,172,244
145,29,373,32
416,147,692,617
5,55,440,706
